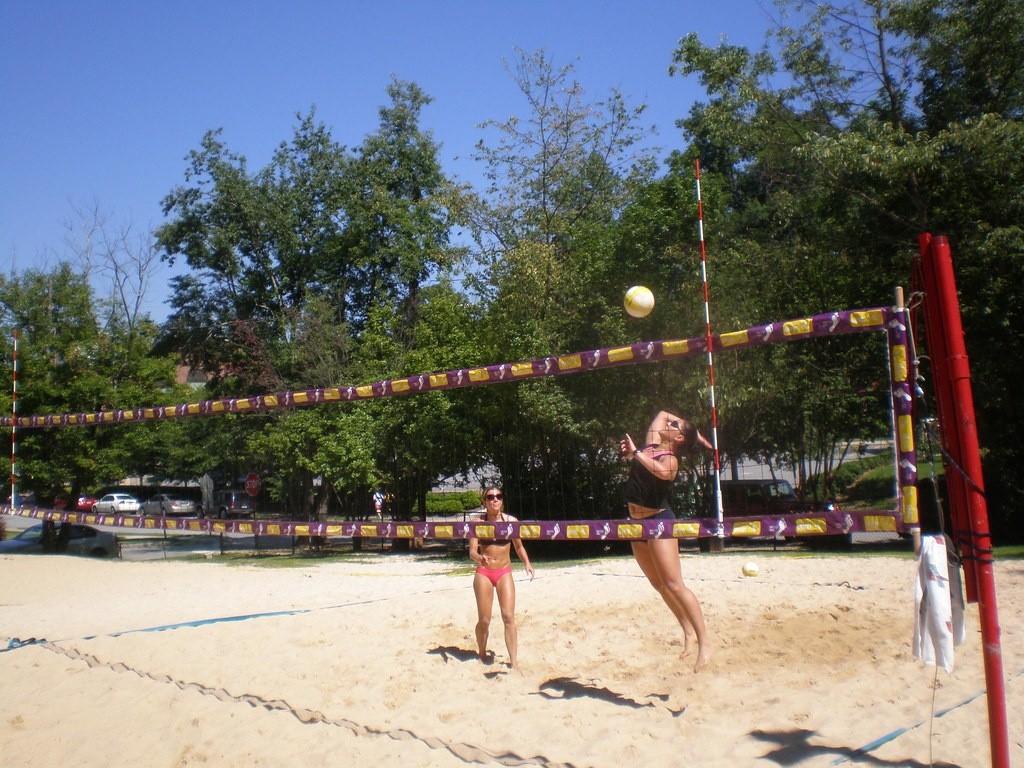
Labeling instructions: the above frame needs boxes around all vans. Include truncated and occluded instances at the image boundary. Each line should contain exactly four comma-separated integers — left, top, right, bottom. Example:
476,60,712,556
711,479,801,511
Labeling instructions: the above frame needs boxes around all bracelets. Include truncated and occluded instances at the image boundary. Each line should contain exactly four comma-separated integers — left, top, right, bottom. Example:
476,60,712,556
633,449,641,457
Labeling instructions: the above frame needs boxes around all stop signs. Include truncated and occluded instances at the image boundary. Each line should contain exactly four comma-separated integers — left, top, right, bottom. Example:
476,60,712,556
244,473,261,496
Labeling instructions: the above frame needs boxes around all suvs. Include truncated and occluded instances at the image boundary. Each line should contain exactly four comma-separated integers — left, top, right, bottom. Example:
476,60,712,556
196,490,253,520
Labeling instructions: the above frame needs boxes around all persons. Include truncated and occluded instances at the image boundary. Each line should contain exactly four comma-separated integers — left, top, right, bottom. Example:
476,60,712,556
620,410,713,672
373,489,395,520
470,487,535,672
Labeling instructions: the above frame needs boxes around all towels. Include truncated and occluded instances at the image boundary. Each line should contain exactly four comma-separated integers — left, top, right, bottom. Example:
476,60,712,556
913,534,967,673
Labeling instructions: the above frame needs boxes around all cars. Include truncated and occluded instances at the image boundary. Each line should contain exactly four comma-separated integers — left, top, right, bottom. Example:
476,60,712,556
92,494,141,513
694,476,715,498
53,494,96,513
140,494,197,516
6,495,30,505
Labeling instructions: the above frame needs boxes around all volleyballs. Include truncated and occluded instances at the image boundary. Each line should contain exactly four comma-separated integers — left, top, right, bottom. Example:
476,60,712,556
624,285,655,318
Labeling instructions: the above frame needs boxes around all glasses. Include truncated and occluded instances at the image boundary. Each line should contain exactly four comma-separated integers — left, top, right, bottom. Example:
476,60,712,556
671,421,686,437
484,494,503,500
